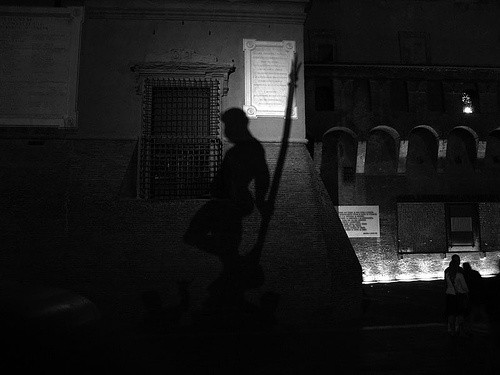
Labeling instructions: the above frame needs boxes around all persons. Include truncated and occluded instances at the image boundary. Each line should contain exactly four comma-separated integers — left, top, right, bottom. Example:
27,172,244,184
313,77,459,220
462,262,483,324
444,254,469,338
